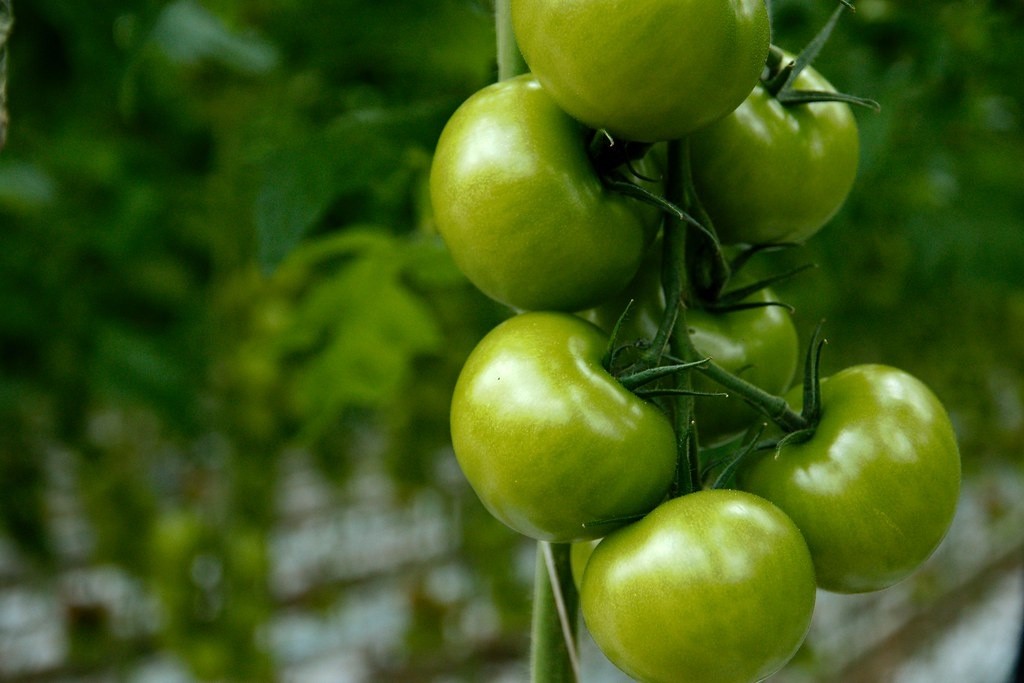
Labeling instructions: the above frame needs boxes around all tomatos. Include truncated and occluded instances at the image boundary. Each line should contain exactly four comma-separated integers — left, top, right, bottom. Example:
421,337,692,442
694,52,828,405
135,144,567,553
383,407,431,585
428,0,964,683
